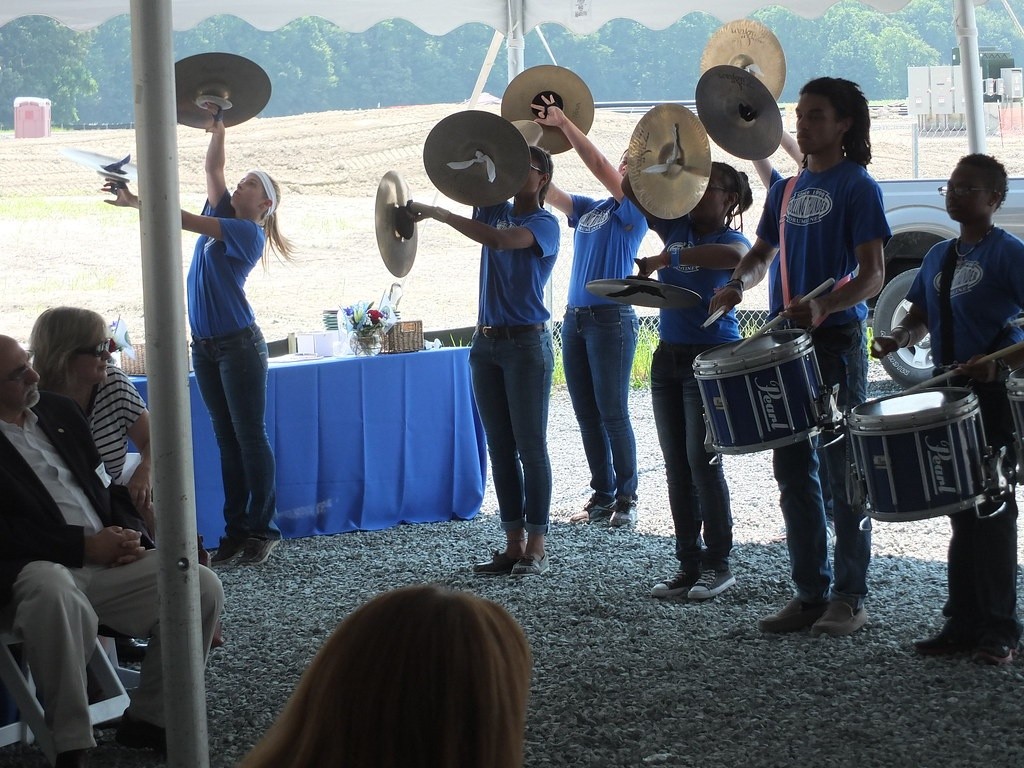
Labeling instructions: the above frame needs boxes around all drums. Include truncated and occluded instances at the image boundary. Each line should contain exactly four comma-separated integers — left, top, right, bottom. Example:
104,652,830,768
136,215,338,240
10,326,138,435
848,388,999,522
691,327,842,456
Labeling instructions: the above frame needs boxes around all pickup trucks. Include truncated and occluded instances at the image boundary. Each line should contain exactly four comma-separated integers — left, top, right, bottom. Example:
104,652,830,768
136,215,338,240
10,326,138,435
875,178,1024,389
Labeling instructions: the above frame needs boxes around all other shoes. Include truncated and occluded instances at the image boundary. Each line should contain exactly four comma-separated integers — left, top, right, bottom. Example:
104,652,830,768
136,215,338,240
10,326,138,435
975,621,1020,664
758,597,829,632
210,533,243,565
115,707,168,756
243,537,273,562
810,602,868,636
912,621,974,656
113,639,147,662
55,748,88,768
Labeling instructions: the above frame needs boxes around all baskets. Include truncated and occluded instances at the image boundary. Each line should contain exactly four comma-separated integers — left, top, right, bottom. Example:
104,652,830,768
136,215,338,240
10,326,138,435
379,320,424,353
120,339,193,377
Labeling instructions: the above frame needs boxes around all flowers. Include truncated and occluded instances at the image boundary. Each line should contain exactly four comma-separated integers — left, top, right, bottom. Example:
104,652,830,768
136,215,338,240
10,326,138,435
341,297,394,331
103,316,136,362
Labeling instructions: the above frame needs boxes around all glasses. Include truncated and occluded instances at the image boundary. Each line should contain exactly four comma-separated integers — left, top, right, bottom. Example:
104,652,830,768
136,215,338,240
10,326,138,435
938,185,1004,199
704,181,735,195
72,339,110,358
0,350,34,384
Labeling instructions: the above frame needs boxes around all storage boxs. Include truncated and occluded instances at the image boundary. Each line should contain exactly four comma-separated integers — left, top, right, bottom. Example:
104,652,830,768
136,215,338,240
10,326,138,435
297,330,339,357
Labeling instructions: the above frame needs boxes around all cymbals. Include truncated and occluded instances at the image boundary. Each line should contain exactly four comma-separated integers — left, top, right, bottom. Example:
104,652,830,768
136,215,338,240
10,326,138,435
702,20,787,101
174,53,272,128
628,105,712,218
376,171,416,277
501,66,595,155
586,279,702,306
423,110,532,207
695,65,782,159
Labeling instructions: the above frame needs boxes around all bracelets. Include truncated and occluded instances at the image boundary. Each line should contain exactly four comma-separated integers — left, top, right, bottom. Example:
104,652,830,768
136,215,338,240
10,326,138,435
670,248,680,267
726,279,744,287
433,206,450,223
892,326,912,349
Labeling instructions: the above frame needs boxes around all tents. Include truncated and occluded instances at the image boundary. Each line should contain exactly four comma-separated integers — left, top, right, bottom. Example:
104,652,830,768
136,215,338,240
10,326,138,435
0,0,908,36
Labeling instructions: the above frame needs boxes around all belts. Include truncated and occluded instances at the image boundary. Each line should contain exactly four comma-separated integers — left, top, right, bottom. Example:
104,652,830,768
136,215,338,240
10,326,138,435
476,322,543,340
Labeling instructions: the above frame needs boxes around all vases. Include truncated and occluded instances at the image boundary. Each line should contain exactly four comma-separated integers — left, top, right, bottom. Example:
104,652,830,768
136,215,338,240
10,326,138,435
350,332,381,356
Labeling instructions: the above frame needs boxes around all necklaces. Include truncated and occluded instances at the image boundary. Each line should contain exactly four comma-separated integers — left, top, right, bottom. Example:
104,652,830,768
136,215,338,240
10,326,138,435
955,224,994,257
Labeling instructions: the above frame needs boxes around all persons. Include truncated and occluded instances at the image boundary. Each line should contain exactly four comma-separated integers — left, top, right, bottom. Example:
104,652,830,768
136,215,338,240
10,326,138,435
232,581,532,768
530,95,647,526
622,162,753,599
101,101,302,565
870,154,1024,664
708,76,893,638
404,147,555,577
0,308,226,768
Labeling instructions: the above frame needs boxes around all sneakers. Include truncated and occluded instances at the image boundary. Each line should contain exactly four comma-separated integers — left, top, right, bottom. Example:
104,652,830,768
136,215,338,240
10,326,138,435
510,553,551,577
609,494,637,525
473,548,517,574
688,568,736,599
570,497,614,524
650,569,697,597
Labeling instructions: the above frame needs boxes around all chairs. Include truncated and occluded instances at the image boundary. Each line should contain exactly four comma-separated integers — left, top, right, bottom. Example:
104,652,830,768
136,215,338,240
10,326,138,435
0,620,131,748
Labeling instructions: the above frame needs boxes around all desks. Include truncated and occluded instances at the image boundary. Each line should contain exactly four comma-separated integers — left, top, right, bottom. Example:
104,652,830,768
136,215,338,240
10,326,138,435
100,348,490,551
